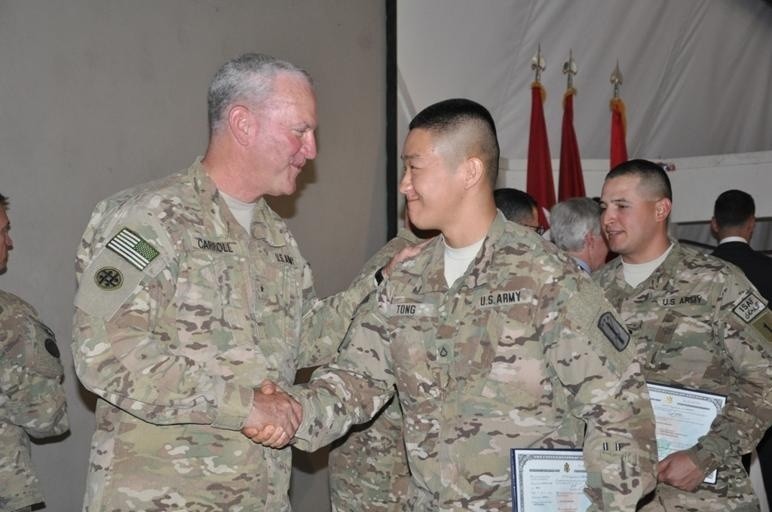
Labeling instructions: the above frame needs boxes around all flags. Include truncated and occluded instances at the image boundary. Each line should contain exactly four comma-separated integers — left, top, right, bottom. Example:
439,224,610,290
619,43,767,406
524,76,559,235
607,93,630,171
554,81,589,201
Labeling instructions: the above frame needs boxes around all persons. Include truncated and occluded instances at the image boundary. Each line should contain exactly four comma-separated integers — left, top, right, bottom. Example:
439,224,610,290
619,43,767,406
585,155,772,512
325,195,446,511
539,194,613,278
485,182,543,240
237,95,663,510
68,50,439,511
0,191,71,512
703,186,772,511
